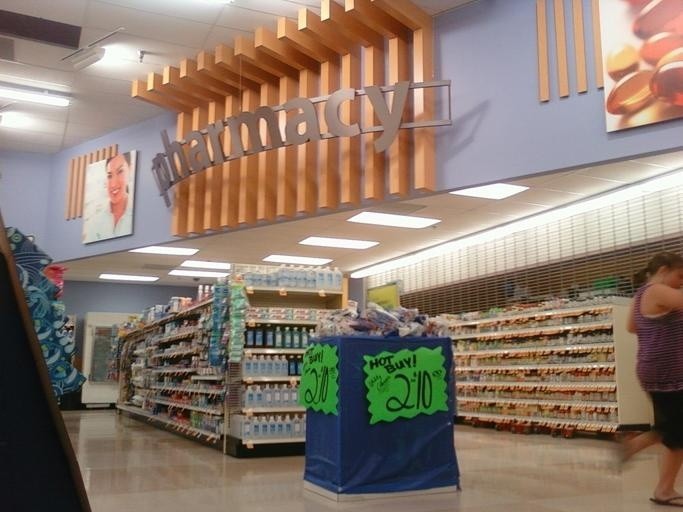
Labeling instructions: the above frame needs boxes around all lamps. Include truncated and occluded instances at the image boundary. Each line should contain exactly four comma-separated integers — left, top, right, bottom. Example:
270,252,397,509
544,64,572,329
0,81,71,106
61,28,124,70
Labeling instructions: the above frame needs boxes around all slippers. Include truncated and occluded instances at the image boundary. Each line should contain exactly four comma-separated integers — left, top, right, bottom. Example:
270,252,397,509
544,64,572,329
649,492,683,507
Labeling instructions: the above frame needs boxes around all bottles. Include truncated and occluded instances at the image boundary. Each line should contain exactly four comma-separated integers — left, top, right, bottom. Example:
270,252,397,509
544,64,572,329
194,284,215,303
243,263,344,293
229,324,319,441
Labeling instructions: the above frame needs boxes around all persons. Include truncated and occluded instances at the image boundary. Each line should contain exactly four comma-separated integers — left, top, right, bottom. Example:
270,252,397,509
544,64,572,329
83,153,132,244
605,249,682,508
